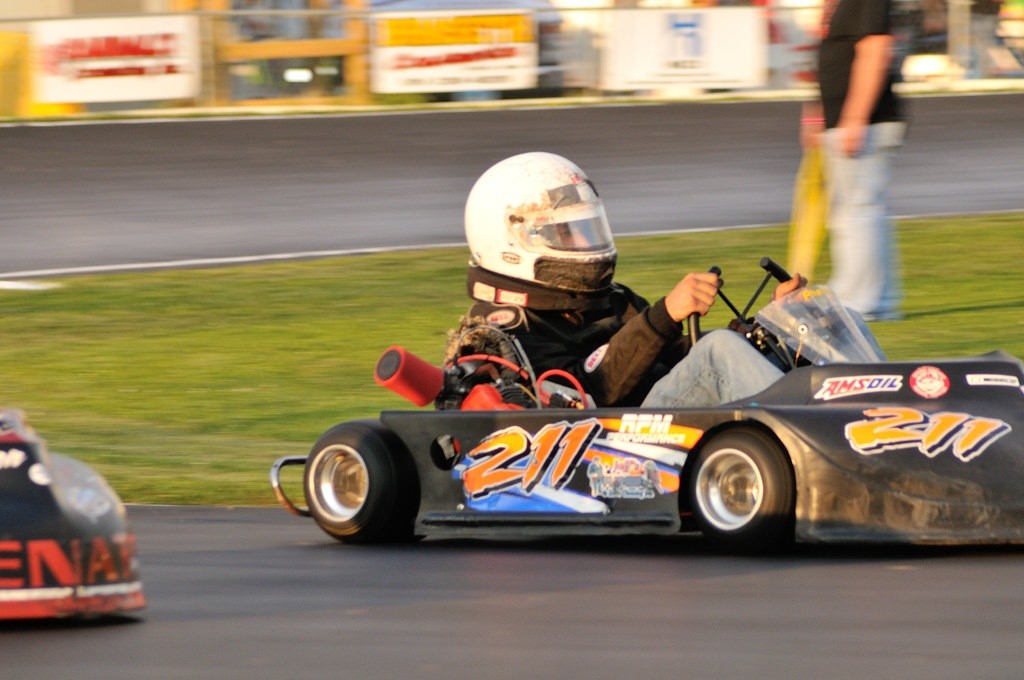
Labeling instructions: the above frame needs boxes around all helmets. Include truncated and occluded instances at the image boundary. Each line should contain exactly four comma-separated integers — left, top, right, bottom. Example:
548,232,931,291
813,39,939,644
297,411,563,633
464,151,617,289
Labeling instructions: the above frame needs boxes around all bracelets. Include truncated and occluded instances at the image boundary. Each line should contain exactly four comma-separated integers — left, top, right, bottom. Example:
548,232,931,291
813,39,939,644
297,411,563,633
801,116,823,126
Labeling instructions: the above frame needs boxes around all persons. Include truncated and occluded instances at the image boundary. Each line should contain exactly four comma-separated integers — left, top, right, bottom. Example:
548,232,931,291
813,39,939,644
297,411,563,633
798,0,907,322
442,152,811,408
